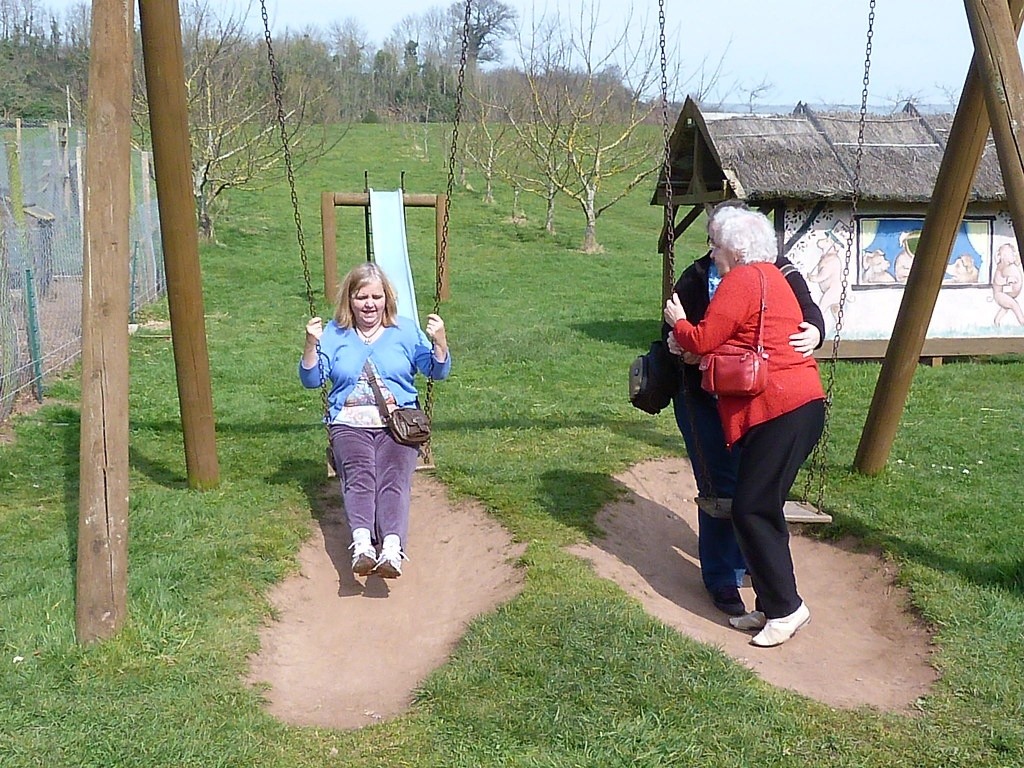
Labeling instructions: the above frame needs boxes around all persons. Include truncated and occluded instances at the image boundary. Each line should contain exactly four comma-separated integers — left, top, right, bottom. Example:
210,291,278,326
299,261,452,579
661,199,826,615
664,206,825,647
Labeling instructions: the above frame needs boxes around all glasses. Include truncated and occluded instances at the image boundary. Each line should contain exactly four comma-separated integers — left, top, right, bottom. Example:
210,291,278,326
708,241,726,252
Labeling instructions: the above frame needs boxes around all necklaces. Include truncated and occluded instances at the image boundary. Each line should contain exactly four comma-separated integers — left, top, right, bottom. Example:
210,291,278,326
356,323,383,344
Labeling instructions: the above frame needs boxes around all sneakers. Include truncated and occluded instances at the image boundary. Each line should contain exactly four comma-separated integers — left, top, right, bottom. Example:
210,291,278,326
346,540,377,577
752,602,811,646
728,610,766,630
373,545,409,579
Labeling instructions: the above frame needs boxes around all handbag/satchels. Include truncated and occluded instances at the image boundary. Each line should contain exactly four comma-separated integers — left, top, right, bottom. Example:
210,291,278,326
387,407,431,445
629,340,680,414
699,343,769,396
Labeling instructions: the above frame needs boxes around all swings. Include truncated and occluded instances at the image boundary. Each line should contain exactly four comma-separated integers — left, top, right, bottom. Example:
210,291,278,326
657,3,874,523
262,0,470,482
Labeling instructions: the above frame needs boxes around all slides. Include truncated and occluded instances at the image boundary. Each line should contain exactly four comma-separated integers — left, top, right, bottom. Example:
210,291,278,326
367,187,421,328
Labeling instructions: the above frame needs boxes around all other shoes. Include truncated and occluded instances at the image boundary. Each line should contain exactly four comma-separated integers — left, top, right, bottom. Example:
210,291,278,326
712,587,746,614
